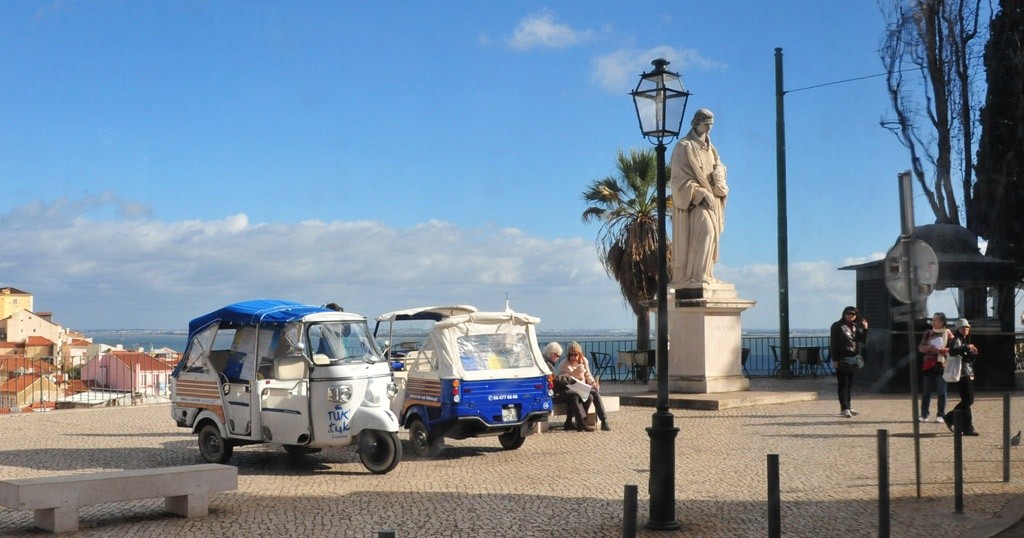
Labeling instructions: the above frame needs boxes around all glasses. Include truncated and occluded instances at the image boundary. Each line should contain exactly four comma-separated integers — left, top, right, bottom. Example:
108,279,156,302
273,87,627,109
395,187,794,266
569,352,580,356
554,353,560,358
846,312,856,316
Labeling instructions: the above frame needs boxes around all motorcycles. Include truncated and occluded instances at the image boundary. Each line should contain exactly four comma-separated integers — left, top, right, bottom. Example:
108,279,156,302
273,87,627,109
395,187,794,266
362,303,554,459
167,298,403,475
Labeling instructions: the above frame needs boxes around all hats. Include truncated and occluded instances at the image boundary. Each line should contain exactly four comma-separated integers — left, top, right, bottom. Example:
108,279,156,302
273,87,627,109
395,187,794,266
956,318,970,329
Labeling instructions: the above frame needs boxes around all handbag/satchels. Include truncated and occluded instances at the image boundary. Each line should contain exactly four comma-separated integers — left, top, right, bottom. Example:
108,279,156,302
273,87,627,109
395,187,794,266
942,339,962,382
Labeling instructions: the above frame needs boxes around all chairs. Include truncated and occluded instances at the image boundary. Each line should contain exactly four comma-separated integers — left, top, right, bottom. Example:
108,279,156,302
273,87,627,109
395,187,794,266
588,351,618,382
633,350,656,381
771,345,835,379
741,348,751,380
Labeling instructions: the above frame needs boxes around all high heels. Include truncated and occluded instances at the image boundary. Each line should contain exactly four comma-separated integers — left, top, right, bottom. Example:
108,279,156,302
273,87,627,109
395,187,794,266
577,423,594,432
564,422,578,430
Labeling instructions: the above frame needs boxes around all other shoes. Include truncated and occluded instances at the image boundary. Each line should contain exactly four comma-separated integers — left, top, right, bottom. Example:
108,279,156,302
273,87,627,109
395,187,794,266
841,409,859,414
843,409,852,417
602,423,610,431
943,415,953,432
935,416,944,422
919,417,929,422
963,431,979,435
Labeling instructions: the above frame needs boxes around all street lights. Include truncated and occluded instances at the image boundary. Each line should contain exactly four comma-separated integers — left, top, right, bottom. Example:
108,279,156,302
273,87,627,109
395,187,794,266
626,58,695,532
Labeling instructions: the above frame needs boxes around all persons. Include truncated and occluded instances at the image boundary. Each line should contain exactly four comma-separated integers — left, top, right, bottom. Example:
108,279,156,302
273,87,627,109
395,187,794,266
671,108,728,285
542,341,611,432
943,318,980,436
830,306,868,417
918,313,954,423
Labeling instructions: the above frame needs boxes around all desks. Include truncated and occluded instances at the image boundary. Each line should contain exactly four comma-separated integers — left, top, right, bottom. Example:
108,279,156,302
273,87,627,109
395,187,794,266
619,349,656,380
792,346,824,377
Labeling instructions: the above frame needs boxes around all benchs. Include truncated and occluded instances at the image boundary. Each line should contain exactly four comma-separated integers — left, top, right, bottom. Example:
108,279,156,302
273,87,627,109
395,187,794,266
0,463,238,533
